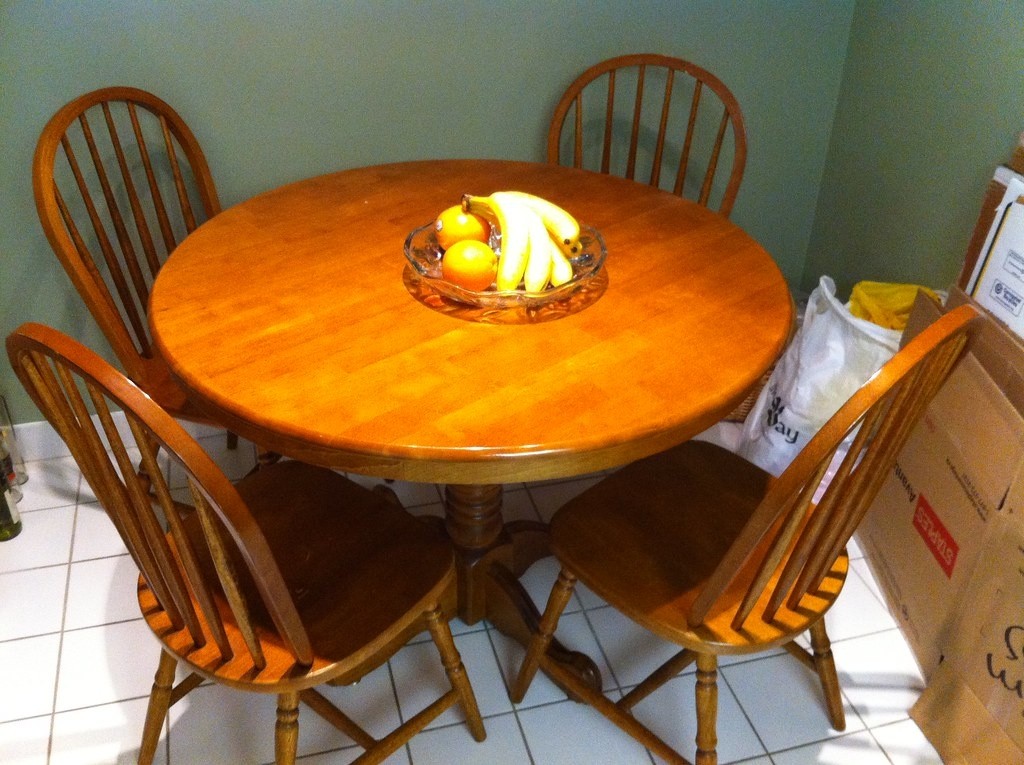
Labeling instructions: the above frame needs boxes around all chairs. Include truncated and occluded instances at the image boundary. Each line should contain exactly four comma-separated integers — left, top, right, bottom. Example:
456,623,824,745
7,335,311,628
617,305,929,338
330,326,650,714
546,51,749,225
509,306,977,765
7,326,487,764
35,85,399,498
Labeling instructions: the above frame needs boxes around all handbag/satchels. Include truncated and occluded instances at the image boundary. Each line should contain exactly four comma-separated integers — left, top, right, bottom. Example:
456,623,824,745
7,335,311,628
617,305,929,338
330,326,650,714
738,275,904,506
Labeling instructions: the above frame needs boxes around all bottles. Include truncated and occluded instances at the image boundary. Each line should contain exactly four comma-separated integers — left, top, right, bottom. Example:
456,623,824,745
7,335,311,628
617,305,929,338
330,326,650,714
0,393,30,544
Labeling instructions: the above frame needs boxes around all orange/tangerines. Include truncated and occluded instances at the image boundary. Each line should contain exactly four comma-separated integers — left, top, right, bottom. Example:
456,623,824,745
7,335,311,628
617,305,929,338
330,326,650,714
442,240,499,292
435,205,491,250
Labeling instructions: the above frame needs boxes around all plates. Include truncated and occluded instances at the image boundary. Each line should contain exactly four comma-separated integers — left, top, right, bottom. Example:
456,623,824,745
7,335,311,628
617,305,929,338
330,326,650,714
402,217,607,308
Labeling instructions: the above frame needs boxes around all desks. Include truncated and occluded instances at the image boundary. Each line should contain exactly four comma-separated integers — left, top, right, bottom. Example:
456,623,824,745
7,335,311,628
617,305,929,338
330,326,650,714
148,159,794,707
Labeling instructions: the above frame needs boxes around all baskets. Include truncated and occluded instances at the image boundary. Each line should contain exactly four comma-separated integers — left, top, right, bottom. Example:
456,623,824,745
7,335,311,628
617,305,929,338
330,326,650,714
723,371,770,423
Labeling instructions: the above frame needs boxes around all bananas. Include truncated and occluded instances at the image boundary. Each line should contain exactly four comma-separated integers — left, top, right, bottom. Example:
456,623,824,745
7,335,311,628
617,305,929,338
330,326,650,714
460,190,581,297
